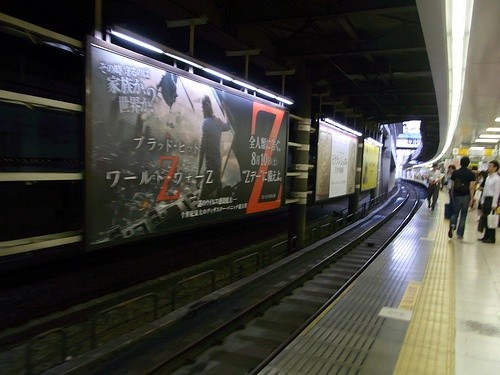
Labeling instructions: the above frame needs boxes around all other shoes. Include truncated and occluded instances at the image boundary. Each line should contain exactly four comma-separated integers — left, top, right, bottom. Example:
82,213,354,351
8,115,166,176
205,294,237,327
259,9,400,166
478,237,487,241
428,203,430,208
483,239,495,243
432,205,434,211
457,235,464,239
448,225,454,238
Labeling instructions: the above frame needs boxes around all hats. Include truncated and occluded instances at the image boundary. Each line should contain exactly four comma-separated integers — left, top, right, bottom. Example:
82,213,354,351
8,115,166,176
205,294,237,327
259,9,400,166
432,163,438,167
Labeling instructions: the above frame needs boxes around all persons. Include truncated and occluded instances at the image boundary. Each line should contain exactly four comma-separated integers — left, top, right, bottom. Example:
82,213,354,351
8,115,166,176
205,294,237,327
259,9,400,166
446,156,475,238
195,95,230,200
477,169,488,210
470,166,479,210
479,161,500,242
448,165,454,202
426,164,442,210
157,72,178,108
416,172,429,184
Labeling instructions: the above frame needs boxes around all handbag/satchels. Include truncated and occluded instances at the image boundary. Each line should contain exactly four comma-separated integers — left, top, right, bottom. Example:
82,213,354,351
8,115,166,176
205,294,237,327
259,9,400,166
487,208,499,228
